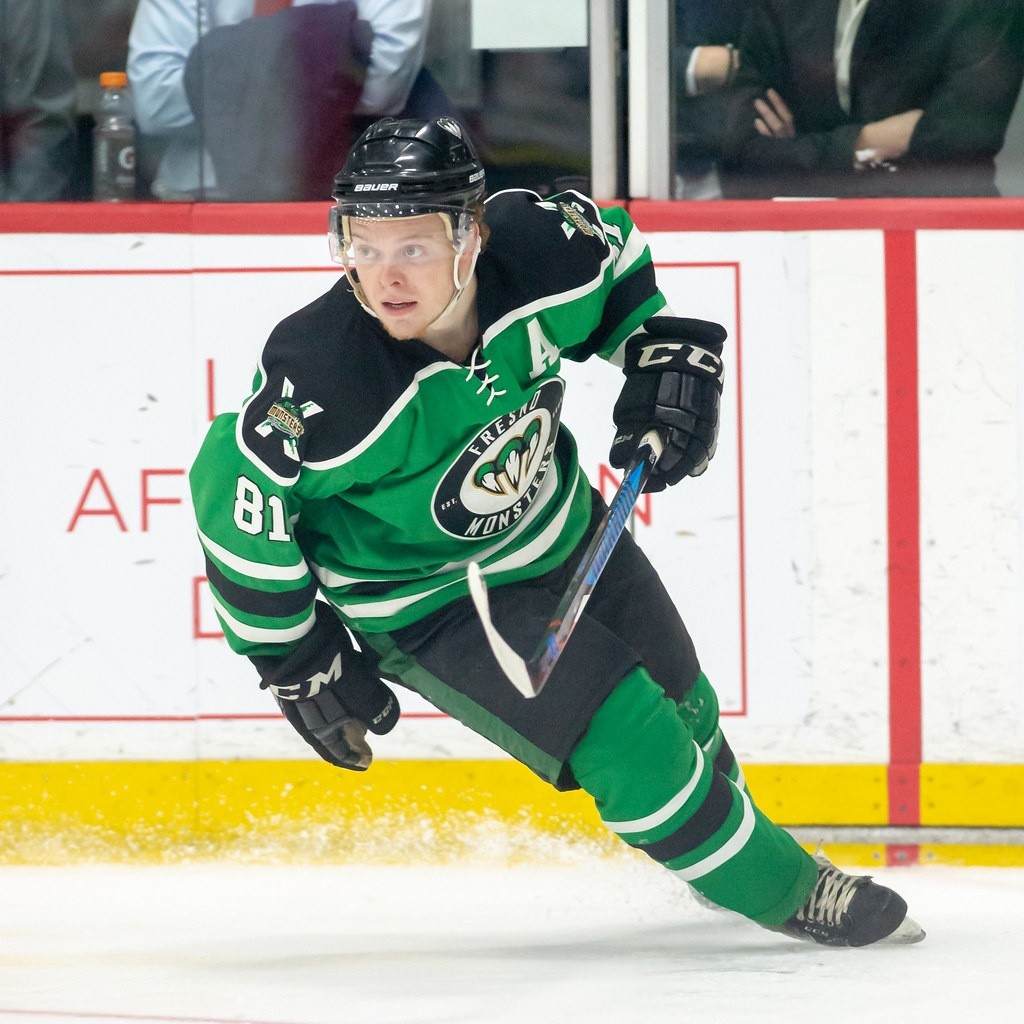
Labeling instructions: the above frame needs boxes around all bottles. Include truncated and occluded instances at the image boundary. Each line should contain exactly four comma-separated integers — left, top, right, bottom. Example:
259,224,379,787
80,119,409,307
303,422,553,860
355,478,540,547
92,72,135,205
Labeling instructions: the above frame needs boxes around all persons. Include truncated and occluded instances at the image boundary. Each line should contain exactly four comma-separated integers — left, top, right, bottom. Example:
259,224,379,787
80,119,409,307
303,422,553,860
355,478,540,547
668,1,1024,199
186,115,914,956
1,0,92,201
124,1,433,200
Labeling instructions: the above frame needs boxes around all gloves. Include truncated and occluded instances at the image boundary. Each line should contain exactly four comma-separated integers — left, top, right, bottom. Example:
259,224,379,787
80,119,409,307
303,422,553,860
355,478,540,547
609,316,726,493
250,600,402,773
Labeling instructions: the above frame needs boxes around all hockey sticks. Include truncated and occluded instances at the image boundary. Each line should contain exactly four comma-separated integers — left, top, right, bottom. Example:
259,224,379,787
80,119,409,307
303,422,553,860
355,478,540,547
468,429,666,700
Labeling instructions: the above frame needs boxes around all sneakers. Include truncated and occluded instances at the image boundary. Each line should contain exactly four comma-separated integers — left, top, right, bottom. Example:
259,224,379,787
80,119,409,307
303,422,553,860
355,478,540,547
781,856,926,952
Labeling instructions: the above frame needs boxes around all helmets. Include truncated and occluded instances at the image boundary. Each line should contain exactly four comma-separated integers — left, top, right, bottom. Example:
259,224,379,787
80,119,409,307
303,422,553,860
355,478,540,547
328,115,487,246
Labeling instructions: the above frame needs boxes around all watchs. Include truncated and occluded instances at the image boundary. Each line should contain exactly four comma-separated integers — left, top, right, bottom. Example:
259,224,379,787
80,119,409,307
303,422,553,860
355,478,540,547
854,146,896,175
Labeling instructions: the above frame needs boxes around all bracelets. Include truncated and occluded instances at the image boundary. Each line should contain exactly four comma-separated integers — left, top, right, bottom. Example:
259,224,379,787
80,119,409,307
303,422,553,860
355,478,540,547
723,41,736,87
